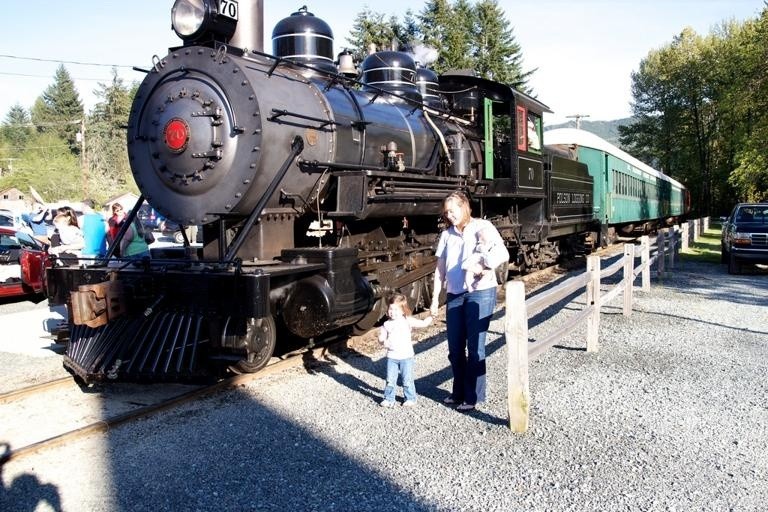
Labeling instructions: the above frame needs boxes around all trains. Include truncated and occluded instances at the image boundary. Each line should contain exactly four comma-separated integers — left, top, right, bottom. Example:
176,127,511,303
46,0,692,391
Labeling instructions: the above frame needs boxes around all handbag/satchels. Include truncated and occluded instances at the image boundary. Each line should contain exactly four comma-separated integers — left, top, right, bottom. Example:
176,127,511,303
144,232,155,246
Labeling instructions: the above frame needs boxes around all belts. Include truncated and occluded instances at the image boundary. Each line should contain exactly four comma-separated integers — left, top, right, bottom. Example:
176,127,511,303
456,401,476,411
444,396,454,404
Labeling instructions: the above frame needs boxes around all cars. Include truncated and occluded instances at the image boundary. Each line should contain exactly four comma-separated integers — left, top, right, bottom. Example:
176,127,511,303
719,203,768,275
0,202,96,302
137,203,203,244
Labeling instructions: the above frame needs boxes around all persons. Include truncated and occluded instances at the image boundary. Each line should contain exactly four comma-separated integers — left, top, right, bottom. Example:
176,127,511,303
121,210,153,260
379,295,438,409
431,192,510,411
77,199,107,260
108,203,127,256
29,204,53,241
54,207,85,255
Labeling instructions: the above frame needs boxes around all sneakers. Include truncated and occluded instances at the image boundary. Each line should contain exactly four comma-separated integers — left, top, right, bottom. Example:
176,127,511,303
379,400,396,408
402,399,419,407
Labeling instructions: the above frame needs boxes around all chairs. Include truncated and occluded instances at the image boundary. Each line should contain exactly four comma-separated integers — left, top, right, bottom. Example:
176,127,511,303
741,213,754,220
